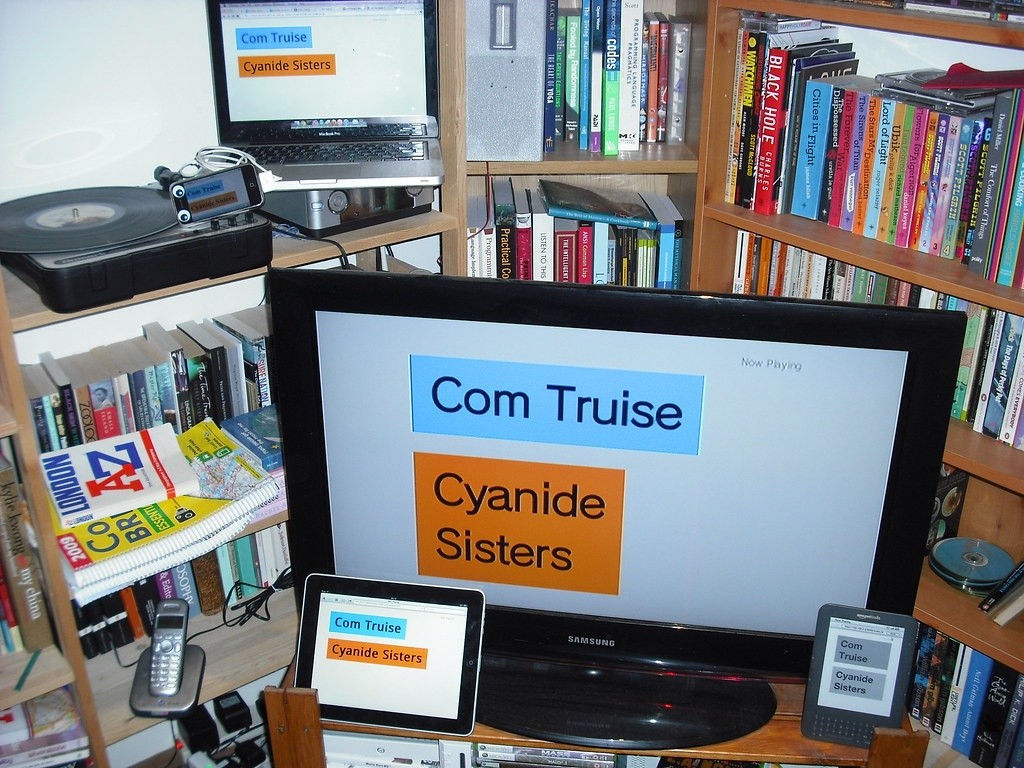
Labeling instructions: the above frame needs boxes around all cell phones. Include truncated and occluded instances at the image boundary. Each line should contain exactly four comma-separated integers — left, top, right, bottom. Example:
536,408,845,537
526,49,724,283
167,165,265,225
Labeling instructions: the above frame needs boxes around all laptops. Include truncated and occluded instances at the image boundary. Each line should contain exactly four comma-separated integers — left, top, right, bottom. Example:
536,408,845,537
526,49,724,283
202,0,444,187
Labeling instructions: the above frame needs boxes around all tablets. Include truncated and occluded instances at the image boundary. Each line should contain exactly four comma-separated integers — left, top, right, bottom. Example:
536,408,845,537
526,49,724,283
289,573,486,735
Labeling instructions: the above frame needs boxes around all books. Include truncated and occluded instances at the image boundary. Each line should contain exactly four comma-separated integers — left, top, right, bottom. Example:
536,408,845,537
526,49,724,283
467,0,1024,452
905,620,1024,768
0,304,292,768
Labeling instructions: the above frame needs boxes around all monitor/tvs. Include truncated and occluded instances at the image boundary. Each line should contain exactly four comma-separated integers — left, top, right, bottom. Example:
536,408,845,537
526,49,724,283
259,268,969,753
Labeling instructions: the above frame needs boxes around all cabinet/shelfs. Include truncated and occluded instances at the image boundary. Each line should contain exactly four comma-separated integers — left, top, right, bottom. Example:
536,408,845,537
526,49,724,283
0,0,1024,768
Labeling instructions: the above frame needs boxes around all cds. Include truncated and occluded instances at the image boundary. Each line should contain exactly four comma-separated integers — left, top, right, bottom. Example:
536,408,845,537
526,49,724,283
928,538,1018,599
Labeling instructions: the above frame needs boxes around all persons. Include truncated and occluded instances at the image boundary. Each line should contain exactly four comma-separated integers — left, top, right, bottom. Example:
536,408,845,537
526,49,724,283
94,388,112,408
